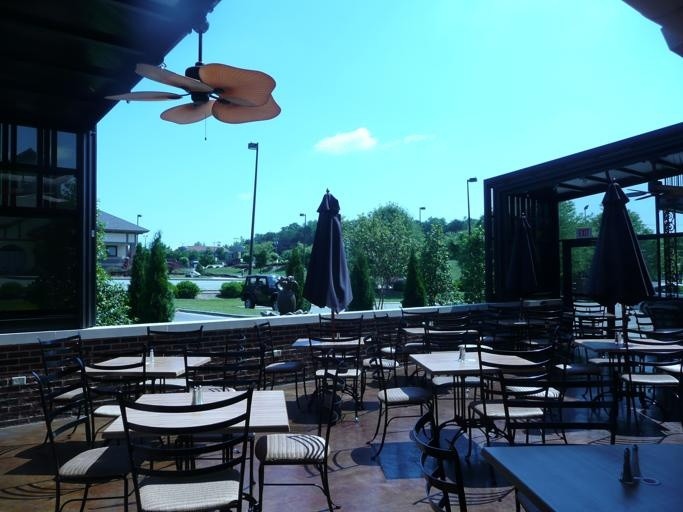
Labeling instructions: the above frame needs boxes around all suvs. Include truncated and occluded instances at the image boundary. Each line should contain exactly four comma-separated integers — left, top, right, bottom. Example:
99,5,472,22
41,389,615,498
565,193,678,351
240,275,279,311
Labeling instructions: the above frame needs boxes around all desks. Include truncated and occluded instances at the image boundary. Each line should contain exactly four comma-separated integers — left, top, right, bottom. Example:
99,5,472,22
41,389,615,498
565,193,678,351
77,356,211,394
103,389,289,512
408,351,545,446
628,328,683,334
480,445,682,512
402,327,478,335
292,336,366,348
573,338,683,408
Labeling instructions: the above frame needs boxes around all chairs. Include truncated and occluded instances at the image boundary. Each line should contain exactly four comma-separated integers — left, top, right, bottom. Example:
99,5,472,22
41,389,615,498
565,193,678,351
457,308,503,352
317,314,364,368
117,383,255,512
573,304,606,340
506,325,573,445
621,329,683,433
374,312,405,371
78,339,147,449
634,312,654,337
37,332,99,446
496,368,621,444
254,320,307,409
400,305,439,376
32,370,136,512
468,343,557,458
519,309,561,352
554,363,602,417
255,356,341,512
578,315,632,398
364,336,433,460
412,406,467,512
139,325,205,390
308,335,362,423
183,330,246,393
631,399,634,415
362,316,406,395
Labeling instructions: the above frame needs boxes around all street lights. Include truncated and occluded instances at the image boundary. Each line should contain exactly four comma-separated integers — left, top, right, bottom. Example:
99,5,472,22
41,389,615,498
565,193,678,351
419,206,426,223
248,143,259,275
137,214,142,225
299,213,308,257
468,178,478,233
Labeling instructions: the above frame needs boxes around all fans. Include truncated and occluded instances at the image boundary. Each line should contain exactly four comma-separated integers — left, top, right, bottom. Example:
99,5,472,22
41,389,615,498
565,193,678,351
104,61,280,139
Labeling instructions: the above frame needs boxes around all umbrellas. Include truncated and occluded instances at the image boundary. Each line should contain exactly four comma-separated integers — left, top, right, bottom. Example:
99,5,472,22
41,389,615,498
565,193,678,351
581,178,656,408
302,189,353,337
